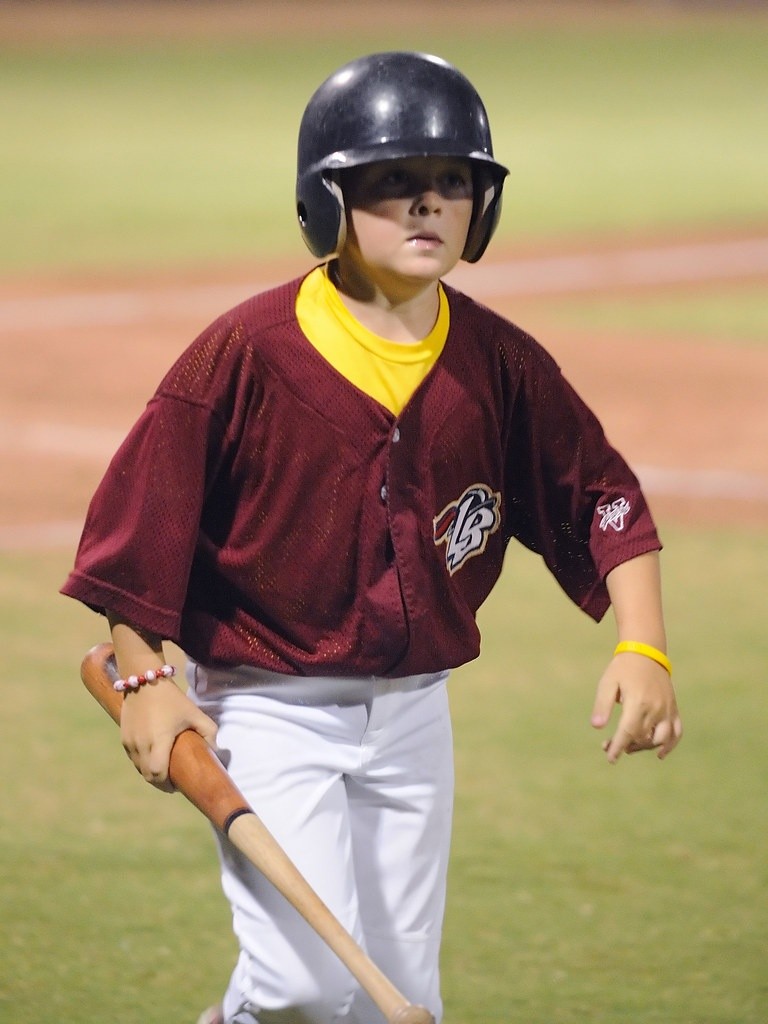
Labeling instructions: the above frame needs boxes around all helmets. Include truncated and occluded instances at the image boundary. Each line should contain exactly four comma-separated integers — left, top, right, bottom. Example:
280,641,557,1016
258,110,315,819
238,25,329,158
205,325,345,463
295,49,512,268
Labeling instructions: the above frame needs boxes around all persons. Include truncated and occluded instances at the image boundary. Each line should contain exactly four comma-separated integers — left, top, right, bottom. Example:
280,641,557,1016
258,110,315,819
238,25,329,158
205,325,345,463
60,50,684,1024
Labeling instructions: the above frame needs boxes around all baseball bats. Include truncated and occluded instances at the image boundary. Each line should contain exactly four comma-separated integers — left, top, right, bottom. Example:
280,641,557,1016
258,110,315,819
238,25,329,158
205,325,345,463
77,640,434,1024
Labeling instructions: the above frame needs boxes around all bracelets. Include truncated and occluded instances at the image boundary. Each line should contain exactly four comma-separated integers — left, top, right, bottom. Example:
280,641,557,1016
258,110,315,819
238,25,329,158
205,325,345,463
613,640,675,676
113,664,177,691
